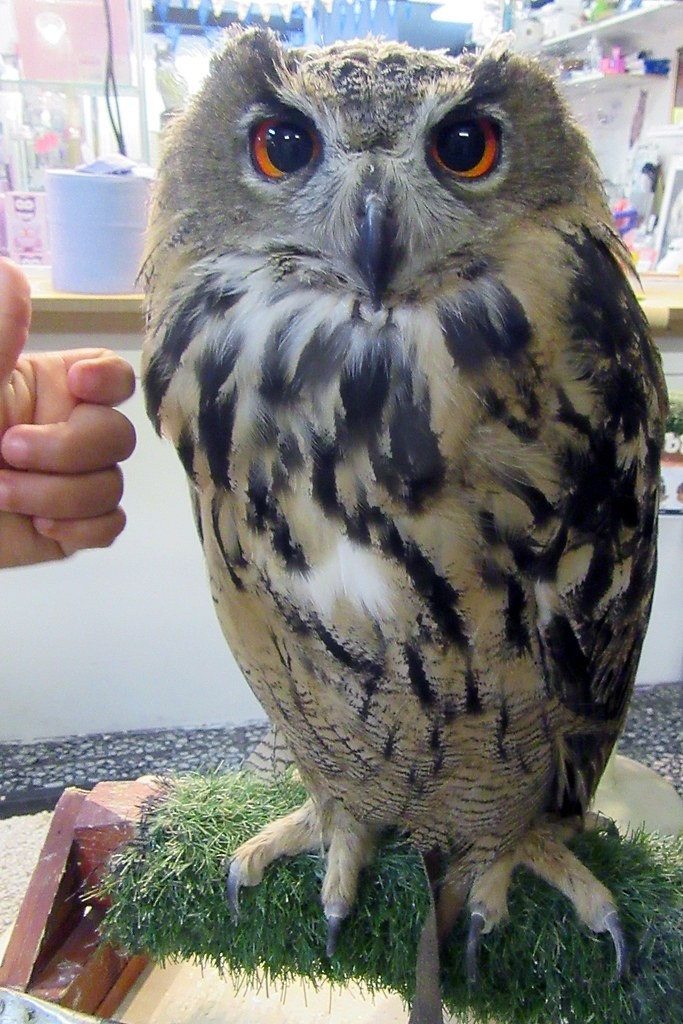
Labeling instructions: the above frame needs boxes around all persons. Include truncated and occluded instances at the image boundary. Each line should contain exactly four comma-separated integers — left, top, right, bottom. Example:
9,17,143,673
0,255,136,570
